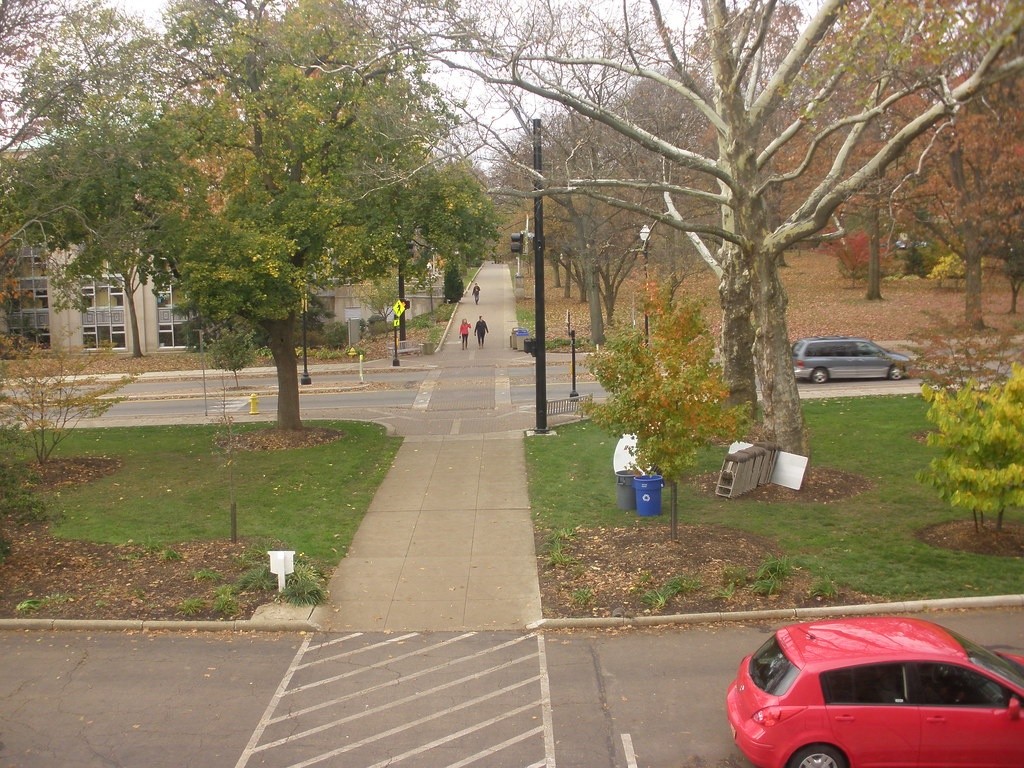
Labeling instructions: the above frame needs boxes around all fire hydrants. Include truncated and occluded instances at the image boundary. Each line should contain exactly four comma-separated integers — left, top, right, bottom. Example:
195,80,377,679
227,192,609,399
347,346,357,360
247,392,261,416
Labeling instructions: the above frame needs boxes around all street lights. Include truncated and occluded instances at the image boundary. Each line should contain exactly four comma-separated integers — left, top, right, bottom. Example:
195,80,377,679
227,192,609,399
638,224,651,356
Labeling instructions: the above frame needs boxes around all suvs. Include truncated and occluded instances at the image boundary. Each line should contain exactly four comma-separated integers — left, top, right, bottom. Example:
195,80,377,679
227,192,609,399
790,334,913,385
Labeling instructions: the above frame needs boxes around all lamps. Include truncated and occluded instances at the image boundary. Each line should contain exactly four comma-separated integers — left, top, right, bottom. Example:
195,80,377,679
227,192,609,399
157,296,166,303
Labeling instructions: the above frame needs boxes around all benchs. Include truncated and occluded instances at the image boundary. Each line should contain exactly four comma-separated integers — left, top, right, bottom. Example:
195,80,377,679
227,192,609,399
385,339,424,359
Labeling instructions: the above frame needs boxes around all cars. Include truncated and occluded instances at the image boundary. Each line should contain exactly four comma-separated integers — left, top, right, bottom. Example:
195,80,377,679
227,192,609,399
724,615,1024,768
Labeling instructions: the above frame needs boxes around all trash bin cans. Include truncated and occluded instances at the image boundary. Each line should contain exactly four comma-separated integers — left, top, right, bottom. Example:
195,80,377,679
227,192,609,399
615,469,643,513
524,338,530,353
516,329,529,350
531,338,537,357
633,474,664,518
510,328,517,350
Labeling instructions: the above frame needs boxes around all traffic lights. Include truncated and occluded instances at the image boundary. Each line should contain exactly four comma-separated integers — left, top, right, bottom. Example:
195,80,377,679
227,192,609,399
400,298,407,310
509,229,524,256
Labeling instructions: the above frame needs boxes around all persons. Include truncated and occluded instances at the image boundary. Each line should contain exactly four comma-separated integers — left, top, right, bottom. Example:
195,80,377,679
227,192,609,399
459,319,471,350
472,283,481,305
474,316,489,348
